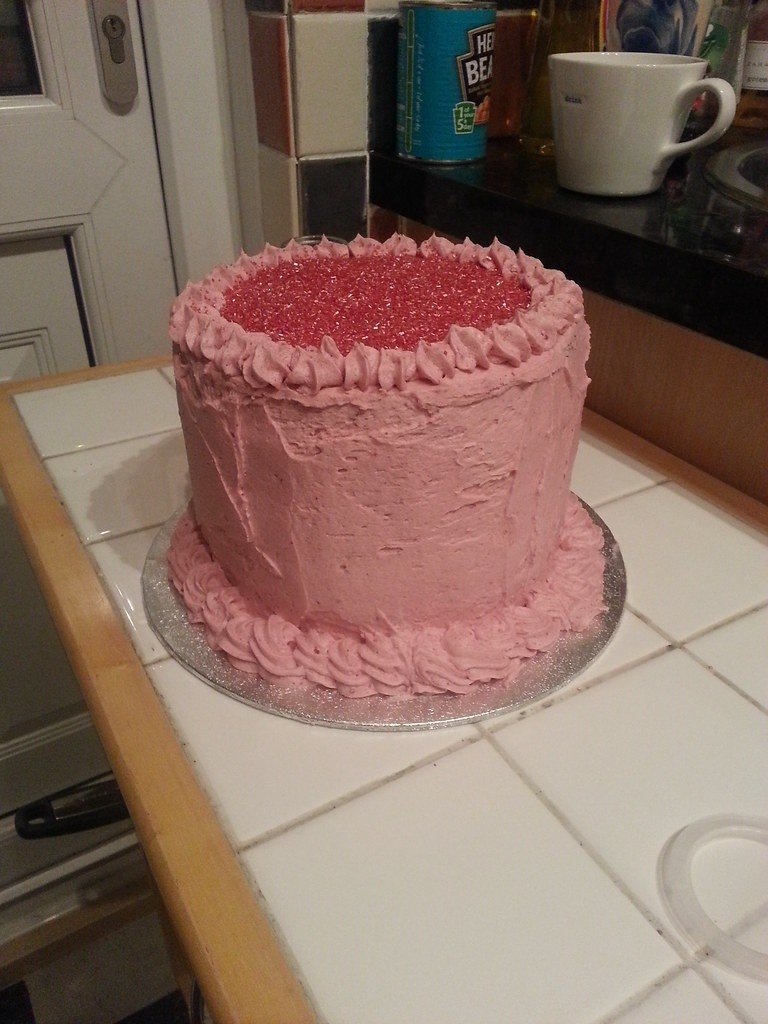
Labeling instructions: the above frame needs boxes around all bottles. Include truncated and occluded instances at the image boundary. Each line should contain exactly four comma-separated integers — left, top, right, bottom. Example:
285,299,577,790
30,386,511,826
699,0,750,93
742,0,768,94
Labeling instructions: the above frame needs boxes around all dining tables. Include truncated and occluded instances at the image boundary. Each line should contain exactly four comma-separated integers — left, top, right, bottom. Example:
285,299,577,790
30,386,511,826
1,355,768,1024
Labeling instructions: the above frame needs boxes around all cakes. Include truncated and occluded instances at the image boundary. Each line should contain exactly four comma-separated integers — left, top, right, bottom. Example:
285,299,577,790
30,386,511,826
164,234,607,701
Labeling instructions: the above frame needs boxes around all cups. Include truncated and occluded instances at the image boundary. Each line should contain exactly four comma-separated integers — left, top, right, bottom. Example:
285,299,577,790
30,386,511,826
547,52,737,195
